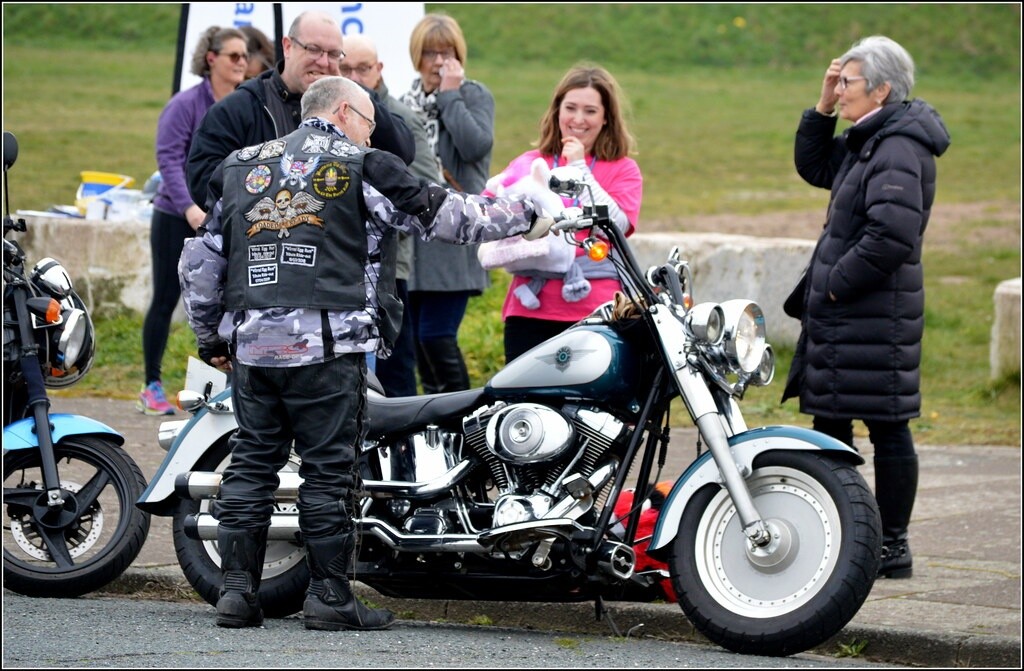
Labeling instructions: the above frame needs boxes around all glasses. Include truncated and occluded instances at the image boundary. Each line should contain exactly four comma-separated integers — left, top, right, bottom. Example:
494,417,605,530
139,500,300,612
290,36,346,62
835,75,867,89
420,49,456,61
217,52,248,64
338,62,378,77
331,104,376,137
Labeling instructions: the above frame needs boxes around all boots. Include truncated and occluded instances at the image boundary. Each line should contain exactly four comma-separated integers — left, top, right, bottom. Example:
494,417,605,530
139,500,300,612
872,453,920,581
215,522,270,627
412,337,471,395
303,529,397,631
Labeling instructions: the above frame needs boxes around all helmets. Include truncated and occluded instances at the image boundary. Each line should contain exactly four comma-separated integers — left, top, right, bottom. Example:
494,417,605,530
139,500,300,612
33,289,97,389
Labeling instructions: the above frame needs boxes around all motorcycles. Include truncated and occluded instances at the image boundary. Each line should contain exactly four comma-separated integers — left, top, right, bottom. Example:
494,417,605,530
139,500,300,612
2,129,152,599
135,174,887,652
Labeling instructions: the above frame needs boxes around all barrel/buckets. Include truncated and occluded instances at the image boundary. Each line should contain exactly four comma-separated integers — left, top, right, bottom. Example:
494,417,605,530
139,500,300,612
75,171,135,217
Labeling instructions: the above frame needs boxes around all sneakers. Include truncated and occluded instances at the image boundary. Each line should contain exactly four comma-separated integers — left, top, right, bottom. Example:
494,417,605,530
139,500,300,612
134,381,175,416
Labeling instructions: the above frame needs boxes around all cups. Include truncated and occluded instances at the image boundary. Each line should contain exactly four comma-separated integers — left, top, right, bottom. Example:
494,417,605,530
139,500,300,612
85,201,105,221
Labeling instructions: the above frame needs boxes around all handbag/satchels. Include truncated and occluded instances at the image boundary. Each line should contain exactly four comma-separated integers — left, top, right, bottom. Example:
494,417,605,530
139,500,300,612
784,266,808,321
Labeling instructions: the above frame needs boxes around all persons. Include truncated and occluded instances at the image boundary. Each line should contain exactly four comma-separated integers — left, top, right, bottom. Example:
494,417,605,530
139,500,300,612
184,9,416,483
395,12,494,398
479,66,641,364
339,32,448,486
232,26,277,81
136,26,250,417
780,36,953,579
176,74,562,628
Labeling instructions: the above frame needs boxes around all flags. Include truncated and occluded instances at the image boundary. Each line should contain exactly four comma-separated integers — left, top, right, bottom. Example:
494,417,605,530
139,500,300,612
172,1,427,102
